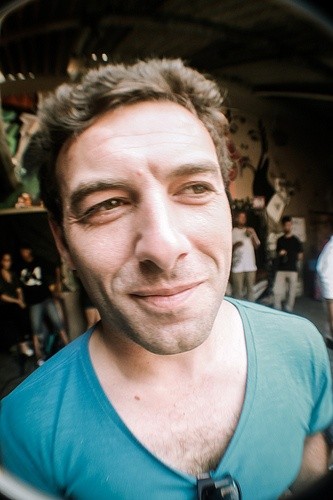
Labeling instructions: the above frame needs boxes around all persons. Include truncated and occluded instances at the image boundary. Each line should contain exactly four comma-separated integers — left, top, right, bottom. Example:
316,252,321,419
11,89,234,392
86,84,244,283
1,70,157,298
0,212,333,369
0,59,333,500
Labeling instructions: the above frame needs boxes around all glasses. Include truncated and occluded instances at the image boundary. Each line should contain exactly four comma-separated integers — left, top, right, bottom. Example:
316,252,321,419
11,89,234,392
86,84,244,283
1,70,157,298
197,472,243,500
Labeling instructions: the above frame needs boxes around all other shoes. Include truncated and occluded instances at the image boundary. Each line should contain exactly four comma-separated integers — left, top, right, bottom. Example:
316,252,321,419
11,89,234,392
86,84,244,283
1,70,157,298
24,349,33,356
37,360,44,367
